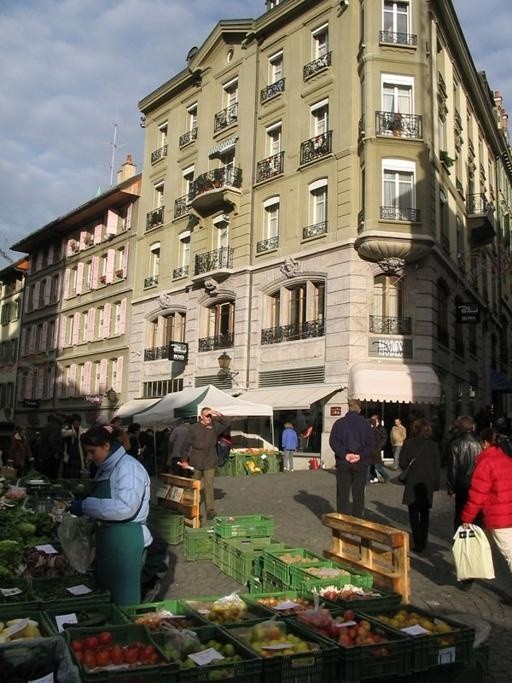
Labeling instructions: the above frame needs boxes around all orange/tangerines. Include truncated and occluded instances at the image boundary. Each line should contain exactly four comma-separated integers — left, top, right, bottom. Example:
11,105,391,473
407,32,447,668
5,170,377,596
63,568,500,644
163,638,242,681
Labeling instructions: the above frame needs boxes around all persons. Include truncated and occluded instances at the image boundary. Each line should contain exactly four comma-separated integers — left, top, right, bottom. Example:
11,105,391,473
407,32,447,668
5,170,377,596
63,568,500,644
10,399,512,606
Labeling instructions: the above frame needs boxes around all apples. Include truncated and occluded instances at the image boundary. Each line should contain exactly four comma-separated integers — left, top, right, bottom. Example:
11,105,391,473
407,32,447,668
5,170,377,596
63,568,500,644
70,631,159,669
208,590,452,657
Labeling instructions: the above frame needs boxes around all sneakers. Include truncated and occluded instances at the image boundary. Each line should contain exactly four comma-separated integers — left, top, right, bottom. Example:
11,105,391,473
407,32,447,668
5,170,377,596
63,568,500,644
206,509,217,519
282,466,295,472
370,477,381,483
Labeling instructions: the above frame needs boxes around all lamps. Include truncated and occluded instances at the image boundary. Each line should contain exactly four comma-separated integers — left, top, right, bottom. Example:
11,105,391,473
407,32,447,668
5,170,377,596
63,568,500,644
217,350,239,386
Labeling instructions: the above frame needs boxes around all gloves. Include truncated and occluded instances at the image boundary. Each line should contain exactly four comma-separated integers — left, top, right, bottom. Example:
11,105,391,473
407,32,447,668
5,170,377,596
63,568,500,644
63,497,84,517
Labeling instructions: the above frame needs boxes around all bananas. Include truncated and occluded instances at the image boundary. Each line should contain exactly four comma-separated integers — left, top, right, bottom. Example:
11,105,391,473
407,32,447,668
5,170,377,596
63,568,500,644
0,618,40,643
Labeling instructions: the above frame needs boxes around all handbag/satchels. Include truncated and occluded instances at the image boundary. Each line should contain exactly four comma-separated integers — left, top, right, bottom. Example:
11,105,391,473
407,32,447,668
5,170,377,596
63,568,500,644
215,439,231,468
0,463,18,479
450,520,497,582
397,464,413,484
